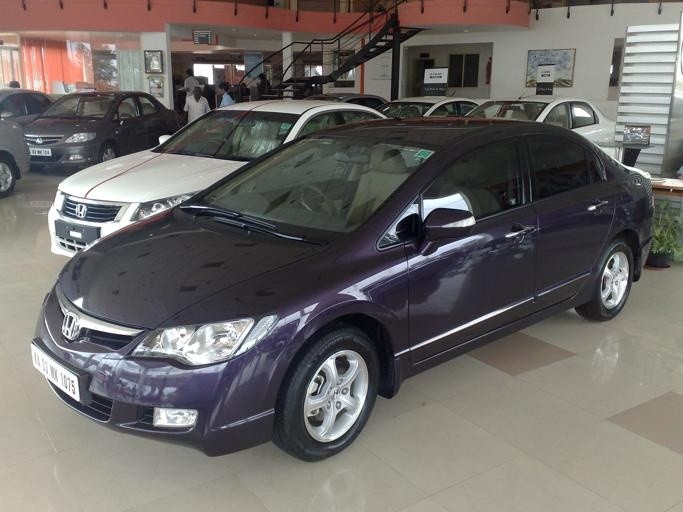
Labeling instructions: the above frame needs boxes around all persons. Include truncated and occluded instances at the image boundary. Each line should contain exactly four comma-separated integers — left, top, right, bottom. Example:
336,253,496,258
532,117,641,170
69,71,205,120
177,68,199,97
181,86,212,127
256,74,269,86
216,82,237,108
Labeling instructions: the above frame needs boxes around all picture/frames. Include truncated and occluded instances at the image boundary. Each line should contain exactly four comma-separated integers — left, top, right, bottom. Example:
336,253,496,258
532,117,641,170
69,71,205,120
525,49,576,88
143,50,163,74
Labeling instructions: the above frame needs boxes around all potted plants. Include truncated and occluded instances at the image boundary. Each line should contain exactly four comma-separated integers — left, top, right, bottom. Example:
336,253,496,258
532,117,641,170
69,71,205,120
645,192,683,271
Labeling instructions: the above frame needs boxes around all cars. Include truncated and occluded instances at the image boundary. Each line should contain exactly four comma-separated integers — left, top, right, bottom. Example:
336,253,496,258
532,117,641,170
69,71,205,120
465,94,627,164
24,91,185,177
304,93,387,110
0,118,29,198
30,115,654,462
358,96,499,118
48,99,396,260
0,87,58,129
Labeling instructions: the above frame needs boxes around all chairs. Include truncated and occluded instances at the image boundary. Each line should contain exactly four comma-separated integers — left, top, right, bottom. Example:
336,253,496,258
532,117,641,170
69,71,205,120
343,144,501,228
75,99,152,119
235,119,282,159
